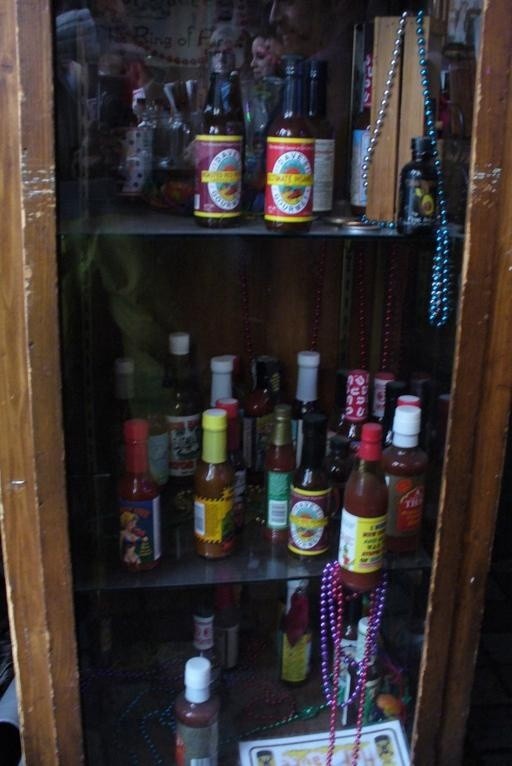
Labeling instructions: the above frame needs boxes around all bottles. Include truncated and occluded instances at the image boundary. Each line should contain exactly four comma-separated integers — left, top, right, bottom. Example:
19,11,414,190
398,137,439,232
194,45,371,232
174,580,386,765
109,329,431,590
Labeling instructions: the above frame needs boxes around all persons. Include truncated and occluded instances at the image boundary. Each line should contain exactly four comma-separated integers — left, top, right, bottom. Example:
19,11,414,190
118,511,146,564
53,1,366,131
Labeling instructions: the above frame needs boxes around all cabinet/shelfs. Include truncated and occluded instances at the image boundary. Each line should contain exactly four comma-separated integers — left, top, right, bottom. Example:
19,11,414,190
2,2,511,763
